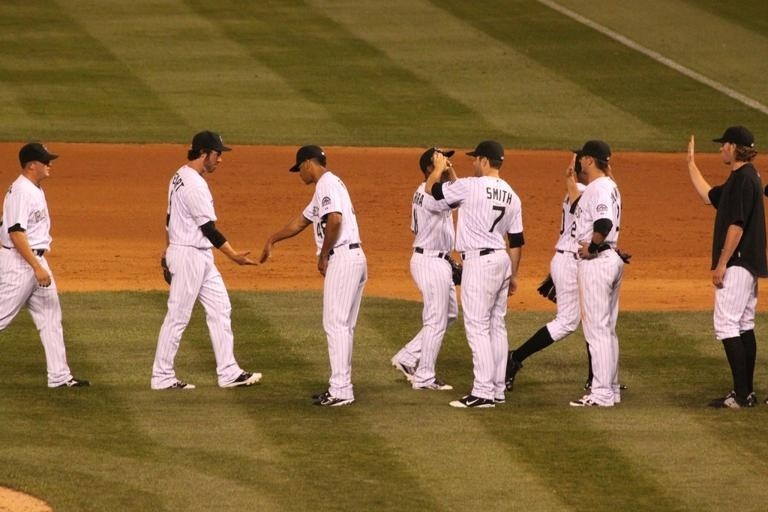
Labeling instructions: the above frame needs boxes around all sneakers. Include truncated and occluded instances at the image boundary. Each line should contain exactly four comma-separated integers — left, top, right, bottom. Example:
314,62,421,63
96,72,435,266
391,356,454,391
312,391,355,407
222,371,262,388
59,377,90,387
167,381,196,390
569,378,628,407
449,351,523,408
707,390,757,408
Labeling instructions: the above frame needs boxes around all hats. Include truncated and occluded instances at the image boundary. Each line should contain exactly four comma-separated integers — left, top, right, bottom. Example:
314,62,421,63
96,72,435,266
713,126,754,148
289,145,326,172
192,130,233,151
570,141,611,161
19,142,59,163
466,141,505,161
420,147,455,172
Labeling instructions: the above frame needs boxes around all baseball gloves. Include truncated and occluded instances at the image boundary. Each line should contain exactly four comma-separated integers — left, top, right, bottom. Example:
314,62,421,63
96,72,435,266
451,262,463,285
162,254,172,285
537,276,555,303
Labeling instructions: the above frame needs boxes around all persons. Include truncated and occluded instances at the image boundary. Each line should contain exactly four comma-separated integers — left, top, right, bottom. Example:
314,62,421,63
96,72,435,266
151,130,263,391
260,144,368,407
389,147,462,390
687,126,768,410
0,143,91,389
425,140,525,409
566,140,624,408
505,151,627,394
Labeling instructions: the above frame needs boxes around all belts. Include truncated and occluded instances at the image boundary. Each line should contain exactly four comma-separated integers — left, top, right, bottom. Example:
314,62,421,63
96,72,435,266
329,244,359,255
462,248,495,260
415,247,452,261
32,249,45,257
596,243,610,253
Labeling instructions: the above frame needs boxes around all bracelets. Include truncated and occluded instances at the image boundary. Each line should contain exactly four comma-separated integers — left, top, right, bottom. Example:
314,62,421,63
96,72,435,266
588,238,601,254
